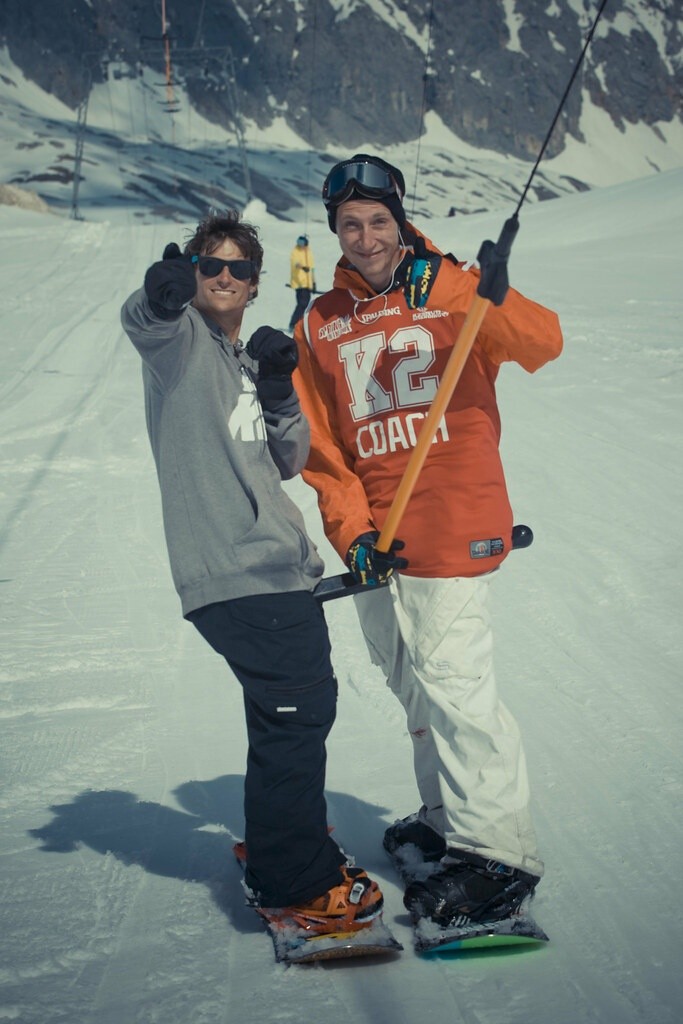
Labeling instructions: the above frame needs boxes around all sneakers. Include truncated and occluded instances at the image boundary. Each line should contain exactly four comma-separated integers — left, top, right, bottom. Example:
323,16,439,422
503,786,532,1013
340,864,368,883
381,811,448,863
402,847,545,928
276,877,384,926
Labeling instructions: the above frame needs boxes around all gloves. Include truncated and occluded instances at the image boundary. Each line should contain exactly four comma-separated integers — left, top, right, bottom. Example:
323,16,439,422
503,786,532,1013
344,530,409,587
477,239,509,306
245,325,299,400
143,242,197,321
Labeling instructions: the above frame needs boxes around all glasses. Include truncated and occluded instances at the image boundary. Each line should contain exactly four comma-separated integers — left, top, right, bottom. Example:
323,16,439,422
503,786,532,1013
321,159,396,207
190,254,258,285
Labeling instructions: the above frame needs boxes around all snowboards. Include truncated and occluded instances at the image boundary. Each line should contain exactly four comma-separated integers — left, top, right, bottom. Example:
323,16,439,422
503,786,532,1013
391,811,552,953
233,827,406,965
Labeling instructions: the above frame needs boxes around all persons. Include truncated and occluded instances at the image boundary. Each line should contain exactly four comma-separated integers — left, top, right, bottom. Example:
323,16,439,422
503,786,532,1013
289,236,316,331
121,208,384,930
291,153,564,929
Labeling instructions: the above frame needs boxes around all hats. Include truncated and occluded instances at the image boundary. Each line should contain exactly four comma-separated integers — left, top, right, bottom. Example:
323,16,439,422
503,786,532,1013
326,154,406,234
